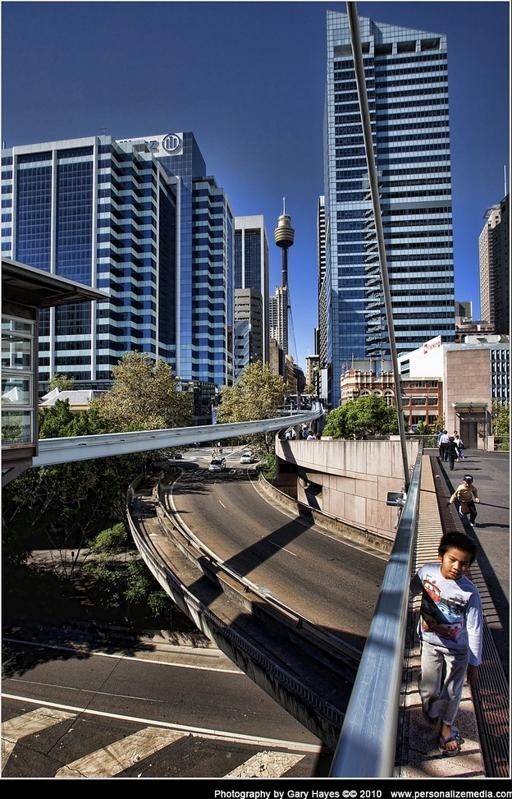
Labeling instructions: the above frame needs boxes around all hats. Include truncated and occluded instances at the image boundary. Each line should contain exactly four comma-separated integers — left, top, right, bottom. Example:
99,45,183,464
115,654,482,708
463,474,473,482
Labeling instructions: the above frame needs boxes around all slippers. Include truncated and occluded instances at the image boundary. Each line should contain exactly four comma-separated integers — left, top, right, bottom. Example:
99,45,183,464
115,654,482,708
439,726,460,757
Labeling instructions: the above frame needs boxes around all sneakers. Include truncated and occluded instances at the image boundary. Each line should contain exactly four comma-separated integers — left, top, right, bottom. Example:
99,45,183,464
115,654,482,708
469,522,474,527
459,505,464,516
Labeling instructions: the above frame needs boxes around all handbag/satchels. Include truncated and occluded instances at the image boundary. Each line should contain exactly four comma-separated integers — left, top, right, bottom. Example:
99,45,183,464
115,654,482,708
459,442,464,449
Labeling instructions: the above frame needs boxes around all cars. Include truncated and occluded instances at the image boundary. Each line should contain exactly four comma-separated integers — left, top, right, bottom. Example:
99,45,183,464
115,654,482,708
209,457,226,473
240,451,254,464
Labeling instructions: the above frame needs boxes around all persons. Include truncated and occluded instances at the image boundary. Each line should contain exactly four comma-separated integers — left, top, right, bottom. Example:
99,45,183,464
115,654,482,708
446,474,481,527
408,529,485,757
437,429,465,471
210,444,224,459
284,422,322,440
